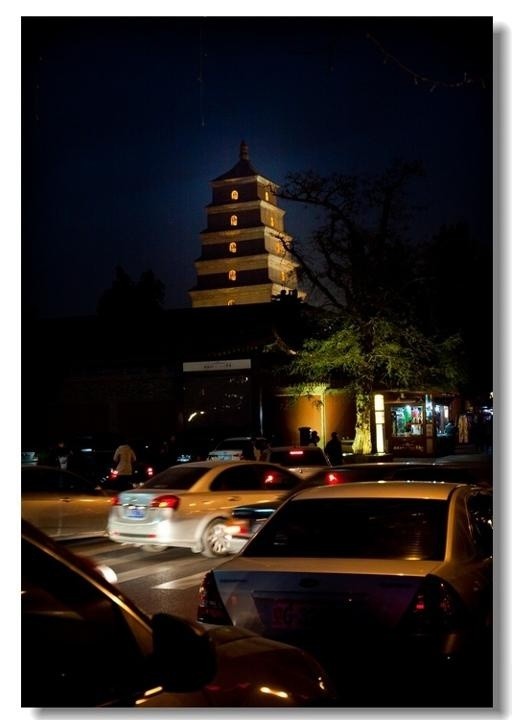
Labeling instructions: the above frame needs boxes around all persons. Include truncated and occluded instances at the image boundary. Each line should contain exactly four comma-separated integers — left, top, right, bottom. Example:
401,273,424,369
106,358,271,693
307,431,320,447
444,421,459,456
324,432,343,467
113,441,136,494
407,420,411,432
239,436,273,463
